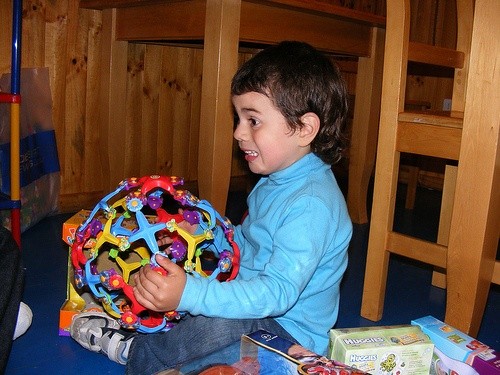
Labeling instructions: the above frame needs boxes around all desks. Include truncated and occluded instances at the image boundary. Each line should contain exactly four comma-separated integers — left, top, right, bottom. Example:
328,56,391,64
78,0,390,227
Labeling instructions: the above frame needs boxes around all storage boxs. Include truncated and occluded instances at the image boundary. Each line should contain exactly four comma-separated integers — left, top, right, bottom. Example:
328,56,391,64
326,315,500,375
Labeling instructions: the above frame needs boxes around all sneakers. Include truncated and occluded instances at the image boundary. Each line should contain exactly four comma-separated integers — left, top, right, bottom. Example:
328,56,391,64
70,312,138,366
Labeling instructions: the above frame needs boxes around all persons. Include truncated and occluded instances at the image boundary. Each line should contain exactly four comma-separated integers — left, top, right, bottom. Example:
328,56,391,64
69,40,354,375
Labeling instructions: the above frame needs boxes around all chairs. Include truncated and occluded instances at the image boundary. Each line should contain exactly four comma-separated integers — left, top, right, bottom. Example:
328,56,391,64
359,0,500,322
